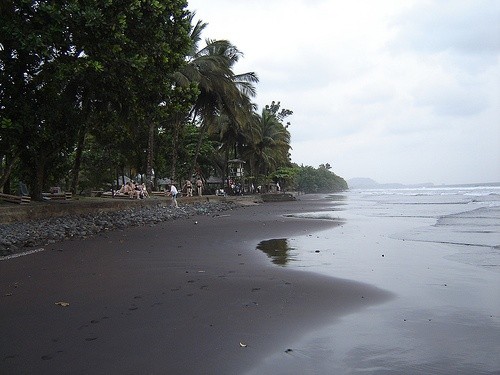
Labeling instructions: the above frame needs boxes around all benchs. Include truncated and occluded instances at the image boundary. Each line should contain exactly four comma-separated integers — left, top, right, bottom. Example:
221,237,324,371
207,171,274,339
0,193,30,204
42,192,65,201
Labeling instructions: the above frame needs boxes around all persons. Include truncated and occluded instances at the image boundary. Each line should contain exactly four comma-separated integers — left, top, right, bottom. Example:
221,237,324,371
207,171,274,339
167,183,180,209
186,179,193,197
230,181,244,197
120,179,151,200
196,177,203,196
